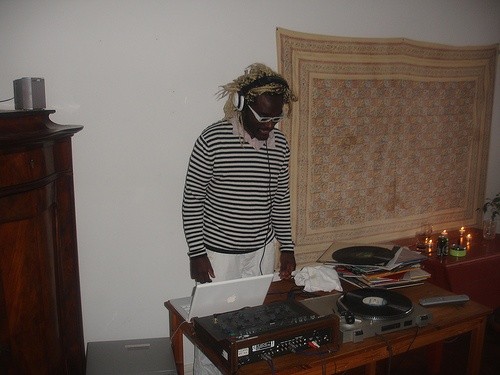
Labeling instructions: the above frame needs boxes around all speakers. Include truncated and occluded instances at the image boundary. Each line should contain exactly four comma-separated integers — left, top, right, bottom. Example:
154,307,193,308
12,78,46,110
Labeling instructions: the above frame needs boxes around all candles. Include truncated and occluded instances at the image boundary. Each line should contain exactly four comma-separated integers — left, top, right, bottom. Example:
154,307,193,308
466,233,472,242
459,227,466,235
441,229,448,235
424,237,433,248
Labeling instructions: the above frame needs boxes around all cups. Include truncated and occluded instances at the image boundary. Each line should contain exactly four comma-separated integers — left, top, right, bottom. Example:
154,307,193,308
482,220,496,240
416,232,428,251
421,223,433,238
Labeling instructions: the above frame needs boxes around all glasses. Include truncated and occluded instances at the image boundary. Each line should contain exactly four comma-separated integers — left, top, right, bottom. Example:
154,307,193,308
245,102,284,122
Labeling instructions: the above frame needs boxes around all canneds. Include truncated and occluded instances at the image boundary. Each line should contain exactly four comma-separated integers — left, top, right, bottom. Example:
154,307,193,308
436,236,448,256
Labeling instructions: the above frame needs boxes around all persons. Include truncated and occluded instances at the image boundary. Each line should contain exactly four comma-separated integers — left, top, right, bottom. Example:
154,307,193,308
182,63,297,375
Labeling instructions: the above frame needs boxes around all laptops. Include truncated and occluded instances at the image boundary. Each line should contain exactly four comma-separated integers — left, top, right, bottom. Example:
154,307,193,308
169,274,274,323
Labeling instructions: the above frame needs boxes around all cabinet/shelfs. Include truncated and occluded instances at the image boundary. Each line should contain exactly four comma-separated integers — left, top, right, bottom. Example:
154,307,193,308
0,110,85,375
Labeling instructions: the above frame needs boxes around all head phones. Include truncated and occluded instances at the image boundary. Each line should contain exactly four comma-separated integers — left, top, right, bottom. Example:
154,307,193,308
232,76,291,111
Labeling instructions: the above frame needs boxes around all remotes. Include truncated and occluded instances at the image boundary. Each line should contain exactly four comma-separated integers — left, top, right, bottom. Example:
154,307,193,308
418,295,470,307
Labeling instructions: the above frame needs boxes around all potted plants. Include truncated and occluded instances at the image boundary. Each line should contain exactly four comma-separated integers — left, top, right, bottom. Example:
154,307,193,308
474,191,500,239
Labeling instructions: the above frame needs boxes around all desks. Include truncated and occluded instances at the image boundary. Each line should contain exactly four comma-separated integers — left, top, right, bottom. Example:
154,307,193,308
163,269,489,375
383,227,500,375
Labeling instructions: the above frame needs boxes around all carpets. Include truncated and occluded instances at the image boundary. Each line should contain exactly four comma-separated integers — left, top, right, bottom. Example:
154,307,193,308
275,26,500,270
